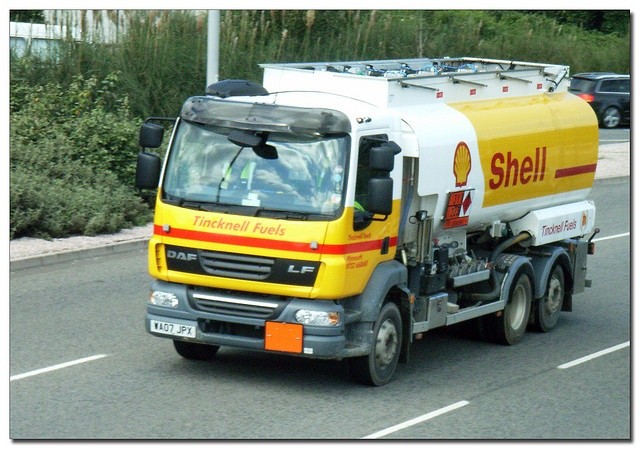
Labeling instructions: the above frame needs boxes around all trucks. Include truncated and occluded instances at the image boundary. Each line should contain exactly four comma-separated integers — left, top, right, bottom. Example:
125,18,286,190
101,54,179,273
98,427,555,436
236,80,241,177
135,59,599,387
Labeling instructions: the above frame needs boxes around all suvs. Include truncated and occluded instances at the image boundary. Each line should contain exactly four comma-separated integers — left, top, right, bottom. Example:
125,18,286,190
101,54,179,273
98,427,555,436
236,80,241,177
569,69,631,129
